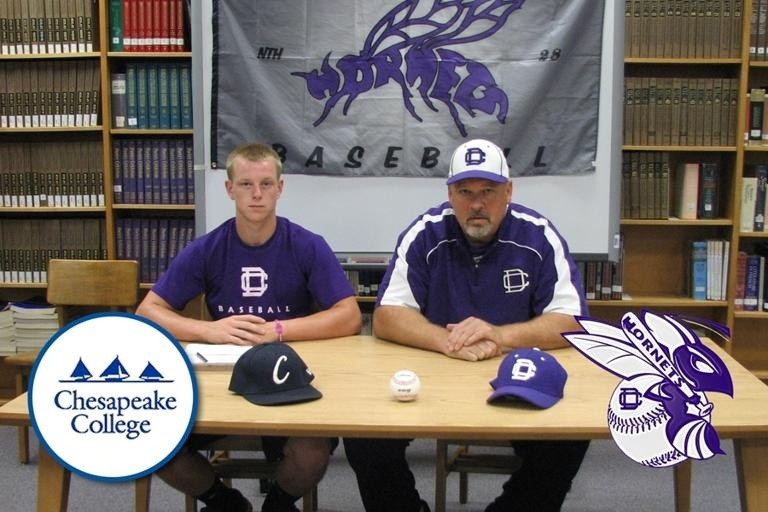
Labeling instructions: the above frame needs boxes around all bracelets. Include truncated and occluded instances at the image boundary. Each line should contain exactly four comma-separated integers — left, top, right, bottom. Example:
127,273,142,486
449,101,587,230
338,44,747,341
274,319,284,342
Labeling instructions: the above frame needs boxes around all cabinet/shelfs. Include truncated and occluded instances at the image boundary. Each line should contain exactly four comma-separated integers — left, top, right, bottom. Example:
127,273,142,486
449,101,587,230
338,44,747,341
0,0,204,399
613,2,768,380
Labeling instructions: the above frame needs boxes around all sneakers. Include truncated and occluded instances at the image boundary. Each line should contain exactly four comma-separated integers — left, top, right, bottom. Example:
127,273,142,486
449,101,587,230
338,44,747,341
199,487,253,512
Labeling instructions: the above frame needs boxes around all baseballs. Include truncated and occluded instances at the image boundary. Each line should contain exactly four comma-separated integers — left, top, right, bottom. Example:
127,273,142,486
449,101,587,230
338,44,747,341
390,371,420,401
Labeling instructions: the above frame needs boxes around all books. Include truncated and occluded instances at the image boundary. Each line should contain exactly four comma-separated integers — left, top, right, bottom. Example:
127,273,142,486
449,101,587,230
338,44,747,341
567,255,621,301
2,4,196,357
338,255,389,296
623,4,768,313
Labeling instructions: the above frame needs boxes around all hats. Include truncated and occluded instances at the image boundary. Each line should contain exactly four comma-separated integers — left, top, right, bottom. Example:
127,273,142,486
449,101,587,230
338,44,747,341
487,347,568,408
445,138,510,185
227,340,322,407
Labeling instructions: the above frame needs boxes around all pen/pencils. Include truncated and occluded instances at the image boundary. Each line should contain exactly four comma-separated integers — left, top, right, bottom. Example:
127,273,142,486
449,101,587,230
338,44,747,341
196,352,208,362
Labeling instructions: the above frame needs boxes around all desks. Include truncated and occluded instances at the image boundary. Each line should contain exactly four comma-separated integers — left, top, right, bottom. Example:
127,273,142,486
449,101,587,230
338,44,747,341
0,336,768,512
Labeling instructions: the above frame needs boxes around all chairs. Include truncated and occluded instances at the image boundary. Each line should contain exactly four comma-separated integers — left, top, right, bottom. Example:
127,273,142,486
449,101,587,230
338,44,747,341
0,258,518,511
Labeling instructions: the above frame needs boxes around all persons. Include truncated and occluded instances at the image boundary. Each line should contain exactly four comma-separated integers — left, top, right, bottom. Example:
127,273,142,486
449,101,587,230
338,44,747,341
135,139,362,512
342,139,592,512
